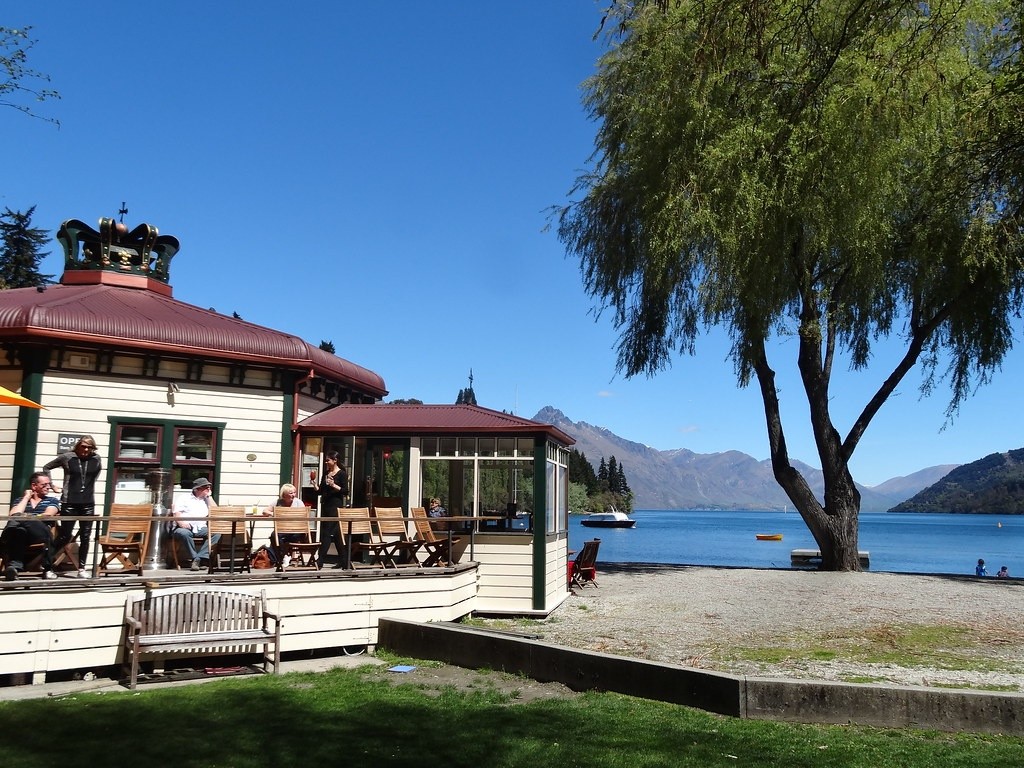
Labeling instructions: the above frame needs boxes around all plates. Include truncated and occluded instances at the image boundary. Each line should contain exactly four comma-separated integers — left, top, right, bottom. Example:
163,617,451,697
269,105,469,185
120,449,144,458
125,436,144,441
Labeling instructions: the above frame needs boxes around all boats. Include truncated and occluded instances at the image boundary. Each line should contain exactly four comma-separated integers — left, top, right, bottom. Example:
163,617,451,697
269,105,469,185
579,511,637,528
756,533,783,540
791,549,871,565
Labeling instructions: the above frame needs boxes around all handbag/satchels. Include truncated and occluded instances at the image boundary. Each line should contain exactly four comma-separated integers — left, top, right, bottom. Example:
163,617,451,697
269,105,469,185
252,549,271,569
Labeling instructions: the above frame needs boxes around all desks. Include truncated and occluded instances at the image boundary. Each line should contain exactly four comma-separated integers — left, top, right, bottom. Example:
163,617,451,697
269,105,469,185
480,511,507,530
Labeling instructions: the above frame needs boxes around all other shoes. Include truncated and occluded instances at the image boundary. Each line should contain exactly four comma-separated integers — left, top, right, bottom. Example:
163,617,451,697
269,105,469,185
191,559,207,570
331,562,346,570
282,555,291,567
4,566,18,580
44,571,57,579
77,570,92,578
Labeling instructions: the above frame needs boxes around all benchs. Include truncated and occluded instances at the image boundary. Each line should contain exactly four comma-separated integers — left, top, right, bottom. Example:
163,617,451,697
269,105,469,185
124,588,281,690
790,549,870,568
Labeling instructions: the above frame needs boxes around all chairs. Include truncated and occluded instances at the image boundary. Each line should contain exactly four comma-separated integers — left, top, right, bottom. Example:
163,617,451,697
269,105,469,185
164,505,460,583
470,502,483,532
0,503,154,577
506,503,523,529
568,539,601,589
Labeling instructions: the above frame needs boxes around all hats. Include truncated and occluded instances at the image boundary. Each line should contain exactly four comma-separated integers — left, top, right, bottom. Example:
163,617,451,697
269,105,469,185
192,477,212,489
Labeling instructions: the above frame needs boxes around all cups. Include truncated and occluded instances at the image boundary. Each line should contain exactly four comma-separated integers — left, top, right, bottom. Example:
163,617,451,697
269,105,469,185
144,453,153,458
206,450,212,460
253,504,258,516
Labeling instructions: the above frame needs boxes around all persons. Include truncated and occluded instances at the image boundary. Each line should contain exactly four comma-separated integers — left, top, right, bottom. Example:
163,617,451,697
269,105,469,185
43,434,102,579
997,566,1008,577
429,498,446,517
166,478,221,570
975,559,989,576
1,472,61,580
310,451,348,569
262,484,306,572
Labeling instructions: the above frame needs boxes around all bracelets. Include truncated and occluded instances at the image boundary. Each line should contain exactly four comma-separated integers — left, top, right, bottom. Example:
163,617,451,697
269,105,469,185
207,495,212,498
